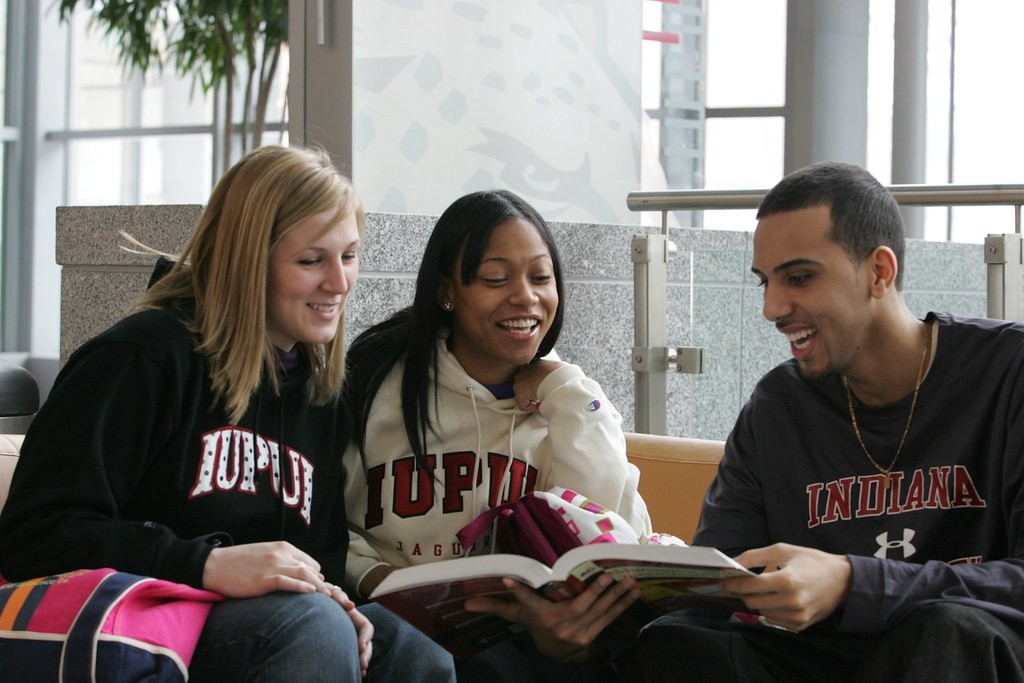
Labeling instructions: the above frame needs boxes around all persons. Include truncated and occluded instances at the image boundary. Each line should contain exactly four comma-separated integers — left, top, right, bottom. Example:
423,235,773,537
0,146,456,683
467,161,1024,683
342,191,652,683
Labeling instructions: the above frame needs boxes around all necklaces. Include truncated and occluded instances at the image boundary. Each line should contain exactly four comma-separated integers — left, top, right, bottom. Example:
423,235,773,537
842,321,930,488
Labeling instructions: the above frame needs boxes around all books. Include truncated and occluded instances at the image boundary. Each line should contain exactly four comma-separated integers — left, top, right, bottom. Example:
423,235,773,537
371,545,758,662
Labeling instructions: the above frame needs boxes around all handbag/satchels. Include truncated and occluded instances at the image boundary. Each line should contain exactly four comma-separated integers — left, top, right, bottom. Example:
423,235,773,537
457,486,686,567
1,566,226,683
